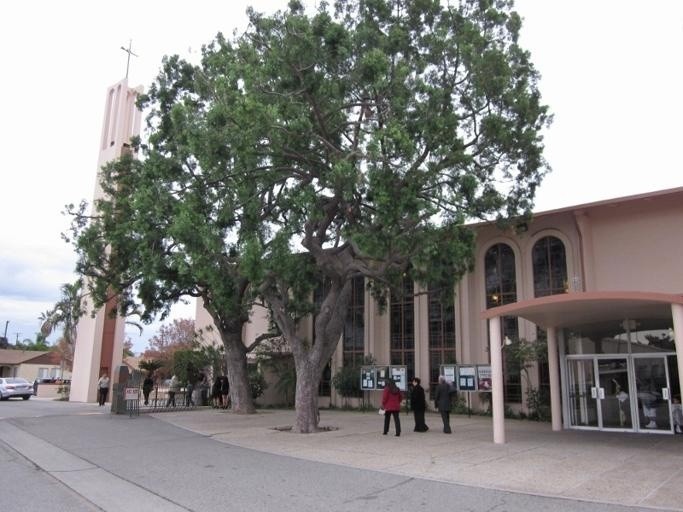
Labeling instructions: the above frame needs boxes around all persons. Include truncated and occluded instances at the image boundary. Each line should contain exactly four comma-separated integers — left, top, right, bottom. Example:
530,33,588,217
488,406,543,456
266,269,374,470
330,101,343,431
616,385,631,427
185,380,195,407
435,376,451,433
212,376,229,409
641,380,658,428
143,375,153,405
98,373,109,406
166,376,178,407
411,378,428,431
672,398,683,433
380,378,404,436
200,377,210,406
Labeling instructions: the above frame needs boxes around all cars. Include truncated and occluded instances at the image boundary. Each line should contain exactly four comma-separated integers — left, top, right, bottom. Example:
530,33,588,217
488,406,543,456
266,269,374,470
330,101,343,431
0,376,36,402
